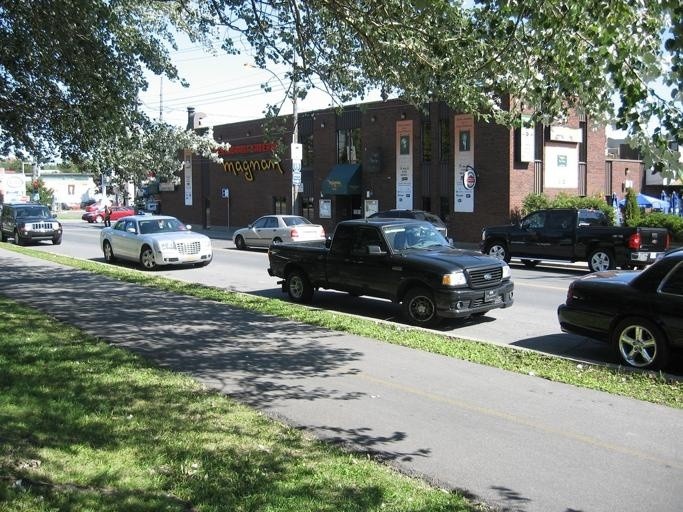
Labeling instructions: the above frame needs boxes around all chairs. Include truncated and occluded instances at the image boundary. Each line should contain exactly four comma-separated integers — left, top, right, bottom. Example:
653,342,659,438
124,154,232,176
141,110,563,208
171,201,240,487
141,221,153,233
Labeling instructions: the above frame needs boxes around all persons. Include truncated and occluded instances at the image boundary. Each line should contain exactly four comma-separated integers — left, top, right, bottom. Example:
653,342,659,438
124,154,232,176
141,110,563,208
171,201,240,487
104,205,111,227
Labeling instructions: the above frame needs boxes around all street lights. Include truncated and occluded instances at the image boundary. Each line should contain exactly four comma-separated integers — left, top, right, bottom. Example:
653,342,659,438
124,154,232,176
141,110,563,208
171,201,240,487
243,63,299,143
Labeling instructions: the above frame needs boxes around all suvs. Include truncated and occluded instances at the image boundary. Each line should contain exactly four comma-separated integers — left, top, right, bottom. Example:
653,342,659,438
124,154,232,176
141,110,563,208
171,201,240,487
365,208,449,244
0,203,63,245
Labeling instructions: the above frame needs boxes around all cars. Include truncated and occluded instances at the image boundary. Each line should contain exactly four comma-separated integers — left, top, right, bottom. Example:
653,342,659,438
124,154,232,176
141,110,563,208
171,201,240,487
100,214,214,271
558,248,682,372
82,200,145,223
233,214,326,251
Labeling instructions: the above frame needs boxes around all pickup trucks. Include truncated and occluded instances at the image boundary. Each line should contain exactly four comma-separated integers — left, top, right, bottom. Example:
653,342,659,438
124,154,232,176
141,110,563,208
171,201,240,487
266,218,514,328
479,207,670,273
